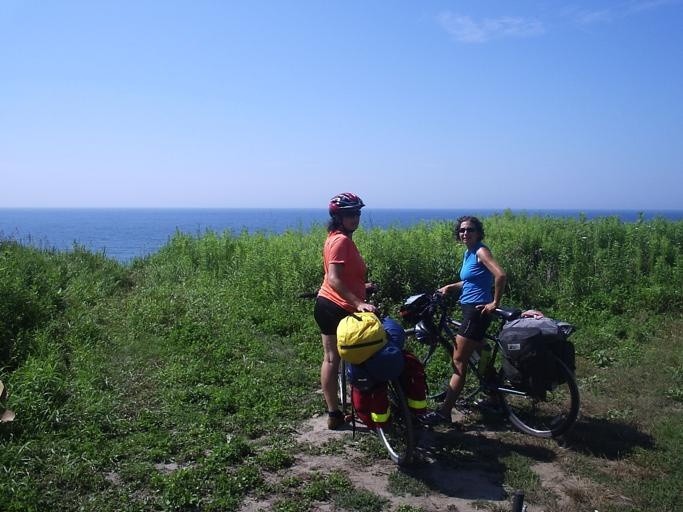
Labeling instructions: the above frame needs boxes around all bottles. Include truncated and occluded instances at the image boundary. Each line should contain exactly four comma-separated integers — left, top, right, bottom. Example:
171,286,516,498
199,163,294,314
479,343,492,376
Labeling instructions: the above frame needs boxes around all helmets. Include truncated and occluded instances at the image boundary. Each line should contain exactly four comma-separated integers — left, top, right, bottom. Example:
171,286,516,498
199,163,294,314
414,319,435,343
329,192,365,218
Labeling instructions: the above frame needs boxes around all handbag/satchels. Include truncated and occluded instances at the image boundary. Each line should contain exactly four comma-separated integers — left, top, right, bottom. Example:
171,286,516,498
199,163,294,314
351,383,390,429
337,311,406,380
498,315,577,394
403,350,427,416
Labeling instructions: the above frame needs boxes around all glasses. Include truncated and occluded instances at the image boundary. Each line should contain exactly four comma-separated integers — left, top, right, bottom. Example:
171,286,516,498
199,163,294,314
341,210,361,218
459,228,475,233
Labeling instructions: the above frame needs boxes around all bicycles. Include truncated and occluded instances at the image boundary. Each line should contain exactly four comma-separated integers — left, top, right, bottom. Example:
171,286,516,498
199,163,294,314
402,287,581,439
298,282,414,466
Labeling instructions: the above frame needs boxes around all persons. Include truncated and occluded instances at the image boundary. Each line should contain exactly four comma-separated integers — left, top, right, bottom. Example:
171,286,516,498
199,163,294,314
416,216,506,425
314,192,380,429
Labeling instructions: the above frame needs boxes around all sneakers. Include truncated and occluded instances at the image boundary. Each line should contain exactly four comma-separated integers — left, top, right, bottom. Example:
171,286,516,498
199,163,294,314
474,396,503,409
418,412,453,425
326,411,345,430
